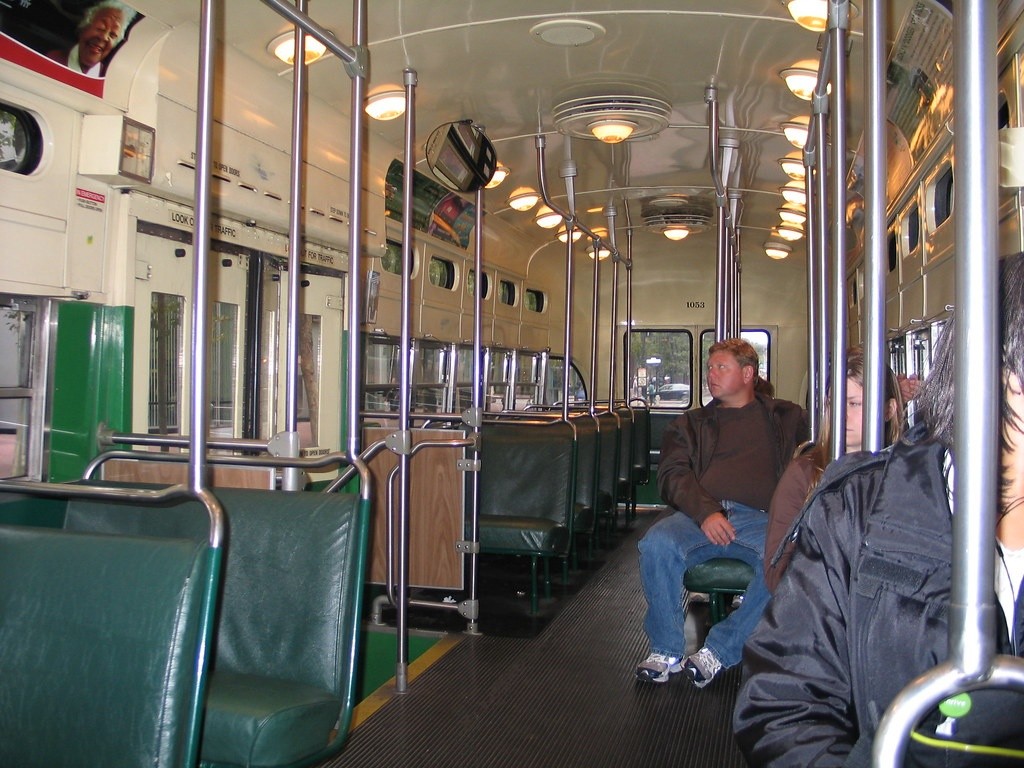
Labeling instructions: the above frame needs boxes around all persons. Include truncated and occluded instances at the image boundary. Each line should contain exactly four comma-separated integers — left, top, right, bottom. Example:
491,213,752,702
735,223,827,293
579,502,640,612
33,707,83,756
896,371,921,405
636,338,820,688
732,250,1024,768
763,343,905,595
48,0,137,79
700,376,776,407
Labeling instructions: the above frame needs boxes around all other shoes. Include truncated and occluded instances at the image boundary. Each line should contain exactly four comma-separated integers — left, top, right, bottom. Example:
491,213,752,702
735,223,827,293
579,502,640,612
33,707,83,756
732,595,744,607
688,591,710,603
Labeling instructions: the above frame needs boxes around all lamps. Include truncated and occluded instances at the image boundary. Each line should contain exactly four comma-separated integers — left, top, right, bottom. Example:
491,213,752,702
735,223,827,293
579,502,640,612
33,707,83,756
266,29,610,260
551,96,672,144
643,213,710,240
764,0,859,260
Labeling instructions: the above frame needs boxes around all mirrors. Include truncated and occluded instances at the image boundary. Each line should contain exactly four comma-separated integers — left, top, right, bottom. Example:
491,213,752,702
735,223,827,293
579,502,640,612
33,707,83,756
426,122,497,193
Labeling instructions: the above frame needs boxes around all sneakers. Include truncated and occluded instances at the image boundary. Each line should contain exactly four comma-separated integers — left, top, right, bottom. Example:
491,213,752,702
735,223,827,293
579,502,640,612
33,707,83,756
635,653,689,683
680,646,722,689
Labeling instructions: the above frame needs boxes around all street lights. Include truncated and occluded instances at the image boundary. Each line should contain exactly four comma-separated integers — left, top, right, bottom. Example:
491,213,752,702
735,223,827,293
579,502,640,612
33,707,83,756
646,356,663,395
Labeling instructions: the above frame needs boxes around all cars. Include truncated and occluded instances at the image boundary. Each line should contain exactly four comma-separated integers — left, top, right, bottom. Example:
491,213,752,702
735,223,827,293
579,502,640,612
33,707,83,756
574,381,588,408
654,383,692,401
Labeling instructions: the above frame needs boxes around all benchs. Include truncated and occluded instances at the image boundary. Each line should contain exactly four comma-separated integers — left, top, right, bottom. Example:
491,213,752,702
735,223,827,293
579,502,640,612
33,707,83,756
0,480,225,768
420,399,651,614
683,559,757,624
65,451,372,768
